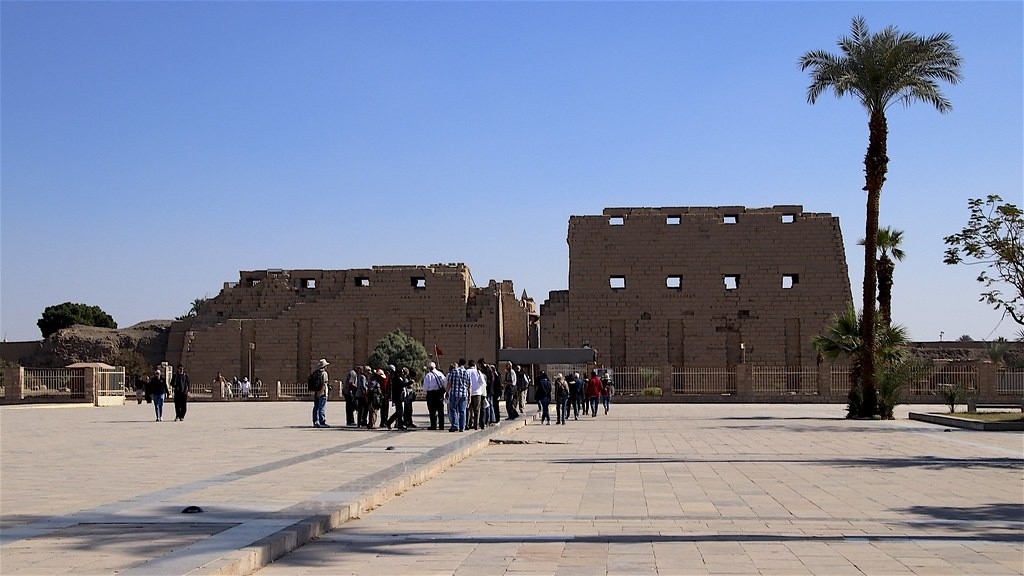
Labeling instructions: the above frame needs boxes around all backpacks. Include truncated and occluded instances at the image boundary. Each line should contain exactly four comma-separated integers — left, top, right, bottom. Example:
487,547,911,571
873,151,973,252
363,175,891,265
558,381,569,397
602,381,613,398
372,388,384,410
308,369,323,391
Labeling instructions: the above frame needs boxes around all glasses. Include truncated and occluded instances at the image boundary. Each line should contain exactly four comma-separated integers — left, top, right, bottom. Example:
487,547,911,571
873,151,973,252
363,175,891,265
155,372,160,375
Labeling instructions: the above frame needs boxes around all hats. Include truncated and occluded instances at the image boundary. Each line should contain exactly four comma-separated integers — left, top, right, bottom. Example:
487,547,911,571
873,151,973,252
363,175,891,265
374,369,386,379
363,365,373,374
317,358,330,369
154,369,161,373
387,364,395,372
553,373,565,380
243,377,248,381
357,365,364,370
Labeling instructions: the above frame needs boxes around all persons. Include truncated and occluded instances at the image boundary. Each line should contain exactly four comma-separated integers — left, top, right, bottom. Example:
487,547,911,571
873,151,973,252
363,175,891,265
344,357,616,433
253,378,260,398
151,370,170,422
313,359,330,428
135,376,144,404
213,372,226,384
144,376,152,403
232,376,242,398
170,364,191,422
225,379,233,398
241,377,251,398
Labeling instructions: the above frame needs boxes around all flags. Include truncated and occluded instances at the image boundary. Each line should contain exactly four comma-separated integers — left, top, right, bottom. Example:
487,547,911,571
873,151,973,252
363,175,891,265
434,345,443,357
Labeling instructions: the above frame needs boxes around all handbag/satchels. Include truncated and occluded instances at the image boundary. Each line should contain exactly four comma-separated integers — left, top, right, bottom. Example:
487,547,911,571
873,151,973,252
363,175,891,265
483,398,490,408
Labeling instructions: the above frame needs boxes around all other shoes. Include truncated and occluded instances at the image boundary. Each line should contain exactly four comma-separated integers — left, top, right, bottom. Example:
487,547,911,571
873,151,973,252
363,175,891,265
137,401,142,404
156,417,163,421
147,401,153,404
312,404,610,432
174,417,185,421
